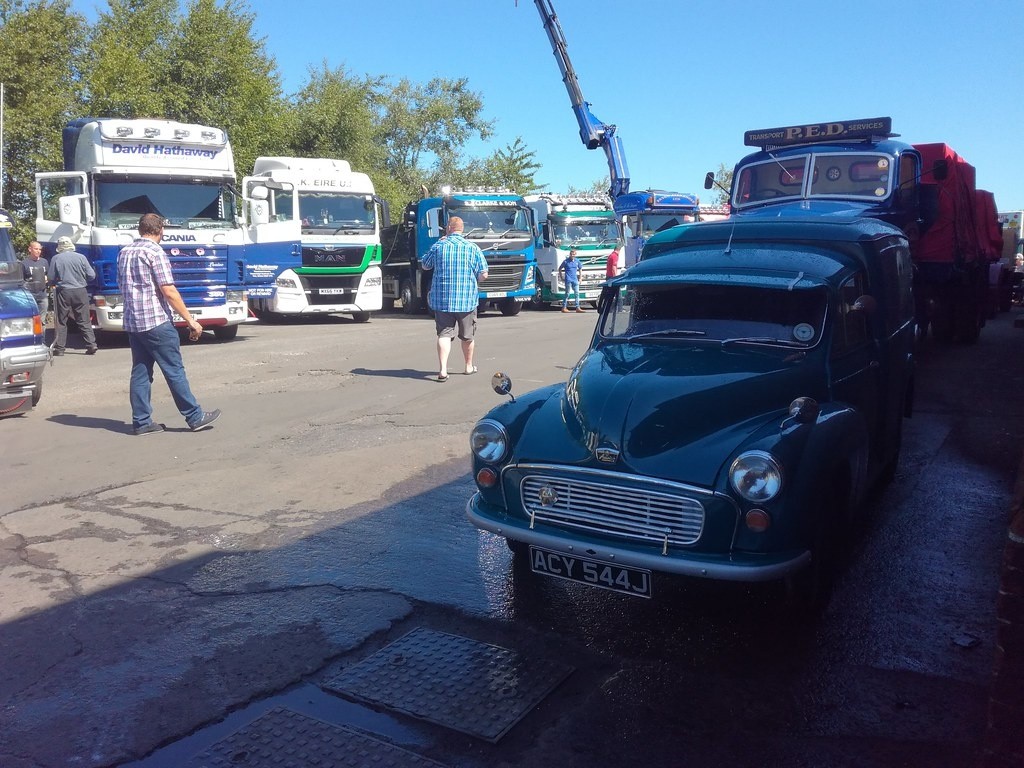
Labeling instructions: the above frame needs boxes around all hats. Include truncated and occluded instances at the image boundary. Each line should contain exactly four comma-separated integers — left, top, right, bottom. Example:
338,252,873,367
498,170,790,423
56,236,75,253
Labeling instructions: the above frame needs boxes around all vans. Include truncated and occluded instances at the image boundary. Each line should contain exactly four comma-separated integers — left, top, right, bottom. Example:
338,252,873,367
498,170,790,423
0,208,48,418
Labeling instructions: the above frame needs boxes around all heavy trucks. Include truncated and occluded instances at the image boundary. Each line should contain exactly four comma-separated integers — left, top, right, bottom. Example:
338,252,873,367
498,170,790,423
378,184,545,317
704,115,1012,356
526,192,630,313
34,118,270,343
234,155,392,323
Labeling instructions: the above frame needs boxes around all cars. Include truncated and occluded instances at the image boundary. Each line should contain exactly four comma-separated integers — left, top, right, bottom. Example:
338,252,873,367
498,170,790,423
464,215,920,622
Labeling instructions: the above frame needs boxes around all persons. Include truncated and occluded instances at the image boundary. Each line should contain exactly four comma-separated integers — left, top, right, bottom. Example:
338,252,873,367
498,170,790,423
606,246,630,313
20,240,49,347
116,213,222,436
558,250,586,313
421,217,488,381
48,236,98,356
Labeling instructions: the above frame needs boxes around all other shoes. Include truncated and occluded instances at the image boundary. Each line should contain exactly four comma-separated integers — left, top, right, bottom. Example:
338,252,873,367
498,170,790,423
562,308,571,313
86,346,98,354
134,424,166,436
576,308,587,313
53,350,65,356
190,409,222,431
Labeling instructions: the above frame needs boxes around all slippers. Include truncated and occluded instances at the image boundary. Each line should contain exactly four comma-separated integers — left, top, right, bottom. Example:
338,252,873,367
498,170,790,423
465,366,478,375
437,374,449,382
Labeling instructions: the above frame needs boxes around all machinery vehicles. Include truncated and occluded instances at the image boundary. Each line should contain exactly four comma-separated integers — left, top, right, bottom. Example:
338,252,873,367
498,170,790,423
525,0,705,274
